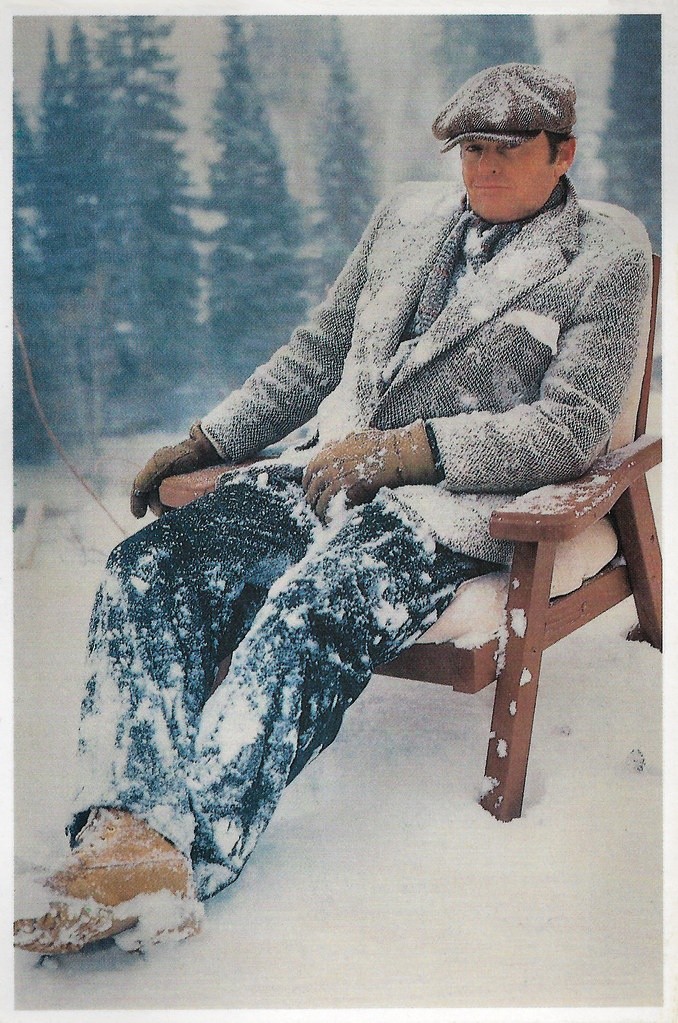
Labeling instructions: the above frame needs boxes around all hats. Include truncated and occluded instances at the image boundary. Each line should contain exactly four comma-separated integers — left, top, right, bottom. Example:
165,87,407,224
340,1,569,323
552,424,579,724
432,62,577,154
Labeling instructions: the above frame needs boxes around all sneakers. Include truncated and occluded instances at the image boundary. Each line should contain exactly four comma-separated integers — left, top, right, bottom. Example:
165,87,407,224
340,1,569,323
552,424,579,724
14,807,203,963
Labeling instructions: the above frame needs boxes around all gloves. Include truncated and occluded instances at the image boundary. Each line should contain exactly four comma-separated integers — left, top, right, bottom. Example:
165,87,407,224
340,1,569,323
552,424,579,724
130,420,224,519
302,418,440,528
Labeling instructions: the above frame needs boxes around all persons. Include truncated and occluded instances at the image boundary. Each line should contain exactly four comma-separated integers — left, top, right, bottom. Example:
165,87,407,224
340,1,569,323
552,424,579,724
14,62,652,955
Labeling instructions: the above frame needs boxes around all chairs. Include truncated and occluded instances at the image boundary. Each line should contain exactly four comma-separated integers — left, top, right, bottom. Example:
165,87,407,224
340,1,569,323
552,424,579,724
150,252,662,822
